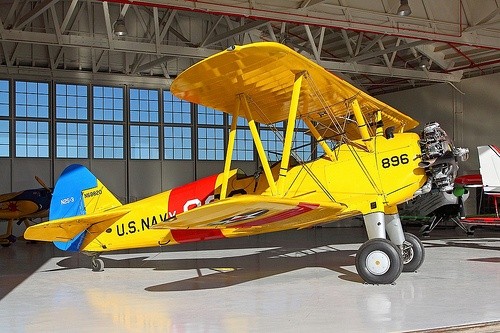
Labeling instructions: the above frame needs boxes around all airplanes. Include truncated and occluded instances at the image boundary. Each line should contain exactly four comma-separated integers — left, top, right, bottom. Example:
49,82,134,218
23,40,469,285
0,175,54,246
404,143,500,239
